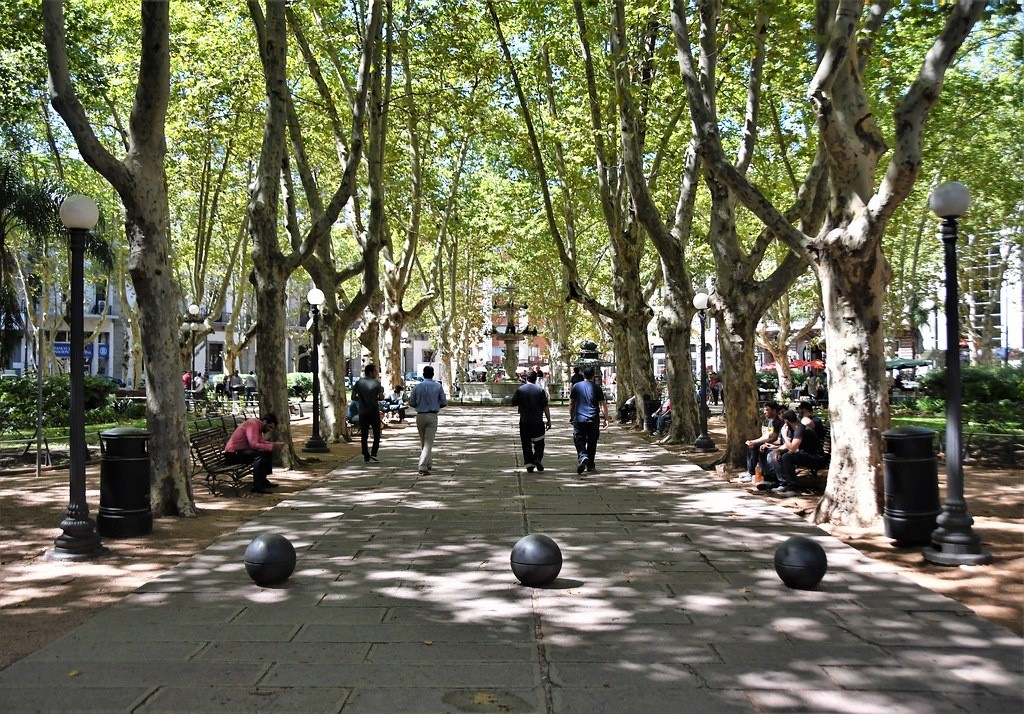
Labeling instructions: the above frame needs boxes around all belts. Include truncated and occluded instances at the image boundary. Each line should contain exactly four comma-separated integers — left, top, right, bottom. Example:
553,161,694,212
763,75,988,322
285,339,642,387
417,411,435,414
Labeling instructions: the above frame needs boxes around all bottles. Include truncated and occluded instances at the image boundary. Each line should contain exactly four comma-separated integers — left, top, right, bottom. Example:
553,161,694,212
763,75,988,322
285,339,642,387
754,462,763,485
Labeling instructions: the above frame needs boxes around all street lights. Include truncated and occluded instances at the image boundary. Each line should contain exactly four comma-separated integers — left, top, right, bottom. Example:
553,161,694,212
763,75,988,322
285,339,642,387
182,304,207,390
399,331,412,381
301,288,331,453
689,293,719,453
51,194,109,560
921,180,994,566
347,333,358,385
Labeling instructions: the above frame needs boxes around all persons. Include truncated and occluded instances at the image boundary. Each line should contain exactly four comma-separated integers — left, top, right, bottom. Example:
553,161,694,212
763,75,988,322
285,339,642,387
610,372,729,444
409,366,448,475
348,385,409,437
569,368,608,473
512,370,552,472
182,370,258,405
225,414,285,494
740,369,831,496
291,380,308,402
453,367,584,400
352,365,385,463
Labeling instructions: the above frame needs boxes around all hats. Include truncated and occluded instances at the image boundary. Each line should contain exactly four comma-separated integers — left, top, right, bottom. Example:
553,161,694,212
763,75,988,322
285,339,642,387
528,370,537,377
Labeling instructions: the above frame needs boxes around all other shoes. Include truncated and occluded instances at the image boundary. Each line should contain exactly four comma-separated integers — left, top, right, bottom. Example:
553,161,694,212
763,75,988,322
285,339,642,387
428,467,431,470
419,470,430,475
400,420,408,424
533,459,543,471
527,467,534,472
653,430,660,435
588,468,595,471
371,455,380,461
757,482,778,491
780,490,797,497
739,470,752,482
764,474,774,481
399,405,408,409
771,485,789,492
251,485,273,494
577,454,588,473
265,479,278,488
364,459,369,464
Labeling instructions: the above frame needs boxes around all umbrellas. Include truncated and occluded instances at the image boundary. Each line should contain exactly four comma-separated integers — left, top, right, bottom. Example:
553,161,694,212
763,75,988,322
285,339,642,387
884,356,932,371
762,359,824,370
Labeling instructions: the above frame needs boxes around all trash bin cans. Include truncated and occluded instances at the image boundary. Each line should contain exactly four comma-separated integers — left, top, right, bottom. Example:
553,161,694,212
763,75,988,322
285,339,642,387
96,428,154,538
232,384,246,400
547,383,562,395
880,427,943,549
643,398,662,431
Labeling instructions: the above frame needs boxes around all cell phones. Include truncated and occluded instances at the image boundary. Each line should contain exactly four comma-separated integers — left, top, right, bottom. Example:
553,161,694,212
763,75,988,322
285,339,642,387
785,420,789,426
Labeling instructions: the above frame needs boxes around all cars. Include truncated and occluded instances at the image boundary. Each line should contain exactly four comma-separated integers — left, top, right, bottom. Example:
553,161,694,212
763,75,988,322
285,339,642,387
1,370,18,380
344,377,350,387
959,349,970,361
406,372,417,379
400,376,421,390
1009,349,1021,357
352,377,360,385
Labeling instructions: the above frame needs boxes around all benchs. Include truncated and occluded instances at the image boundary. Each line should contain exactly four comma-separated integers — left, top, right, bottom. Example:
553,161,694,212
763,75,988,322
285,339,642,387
287,388,308,402
189,426,256,498
627,405,637,423
761,426,831,490
346,419,362,436
